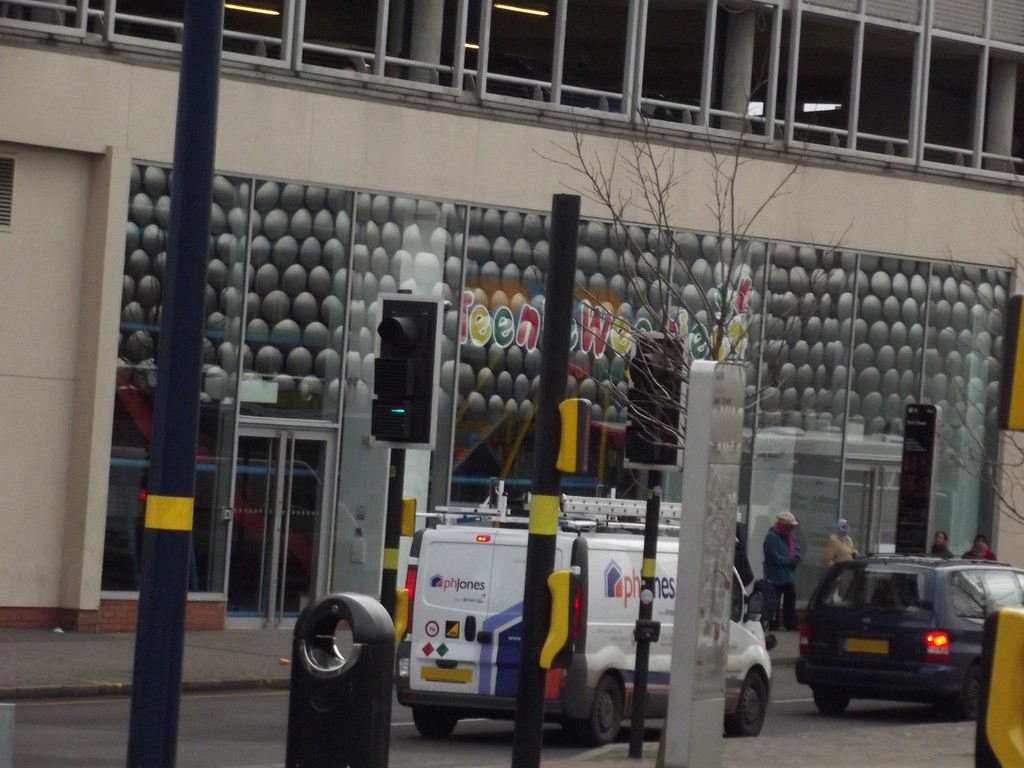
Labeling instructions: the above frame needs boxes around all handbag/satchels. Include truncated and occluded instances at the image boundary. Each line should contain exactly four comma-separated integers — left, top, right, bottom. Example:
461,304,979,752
846,538,858,559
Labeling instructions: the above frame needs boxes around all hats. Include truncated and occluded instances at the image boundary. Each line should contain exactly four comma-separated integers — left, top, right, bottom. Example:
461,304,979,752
776,512,798,525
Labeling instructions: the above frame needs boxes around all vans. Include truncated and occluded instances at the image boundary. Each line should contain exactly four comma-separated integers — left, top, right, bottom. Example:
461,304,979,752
389,495,774,745
796,551,1024,723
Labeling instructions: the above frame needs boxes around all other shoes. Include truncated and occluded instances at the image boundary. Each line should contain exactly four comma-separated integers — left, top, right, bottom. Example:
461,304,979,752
785,624,801,631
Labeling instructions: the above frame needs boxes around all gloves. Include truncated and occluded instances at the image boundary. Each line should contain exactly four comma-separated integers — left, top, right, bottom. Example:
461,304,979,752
785,560,797,571
795,556,800,563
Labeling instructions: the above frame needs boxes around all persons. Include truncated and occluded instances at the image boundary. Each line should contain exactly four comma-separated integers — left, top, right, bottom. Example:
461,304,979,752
825,518,861,568
930,530,955,560
762,511,803,633
961,533,998,561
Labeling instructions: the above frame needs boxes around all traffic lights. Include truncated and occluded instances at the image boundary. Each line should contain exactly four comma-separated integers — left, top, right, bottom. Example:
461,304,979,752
625,335,689,475
368,292,441,453
